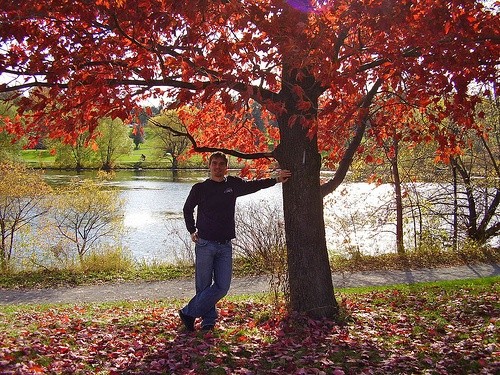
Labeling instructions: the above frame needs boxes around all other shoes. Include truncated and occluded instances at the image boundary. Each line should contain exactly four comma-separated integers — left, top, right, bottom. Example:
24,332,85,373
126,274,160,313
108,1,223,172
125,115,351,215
202,318,215,328
178,310,196,331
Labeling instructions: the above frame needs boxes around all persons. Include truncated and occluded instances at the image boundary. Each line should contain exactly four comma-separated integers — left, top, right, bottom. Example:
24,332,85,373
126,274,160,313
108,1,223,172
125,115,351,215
178,152,291,328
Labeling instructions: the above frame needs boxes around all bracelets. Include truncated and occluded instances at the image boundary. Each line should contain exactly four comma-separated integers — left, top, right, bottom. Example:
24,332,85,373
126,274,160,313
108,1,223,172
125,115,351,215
275,177,280,183
